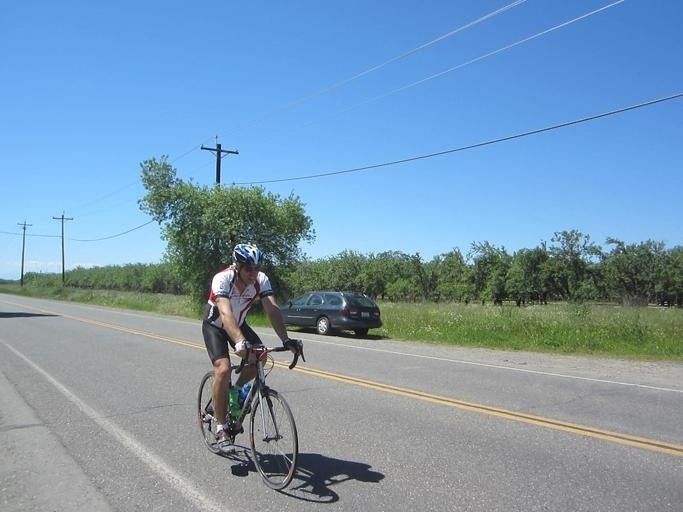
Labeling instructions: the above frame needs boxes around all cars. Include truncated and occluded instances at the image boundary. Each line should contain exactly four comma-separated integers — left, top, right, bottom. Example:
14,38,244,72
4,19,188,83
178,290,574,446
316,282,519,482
279,291,382,338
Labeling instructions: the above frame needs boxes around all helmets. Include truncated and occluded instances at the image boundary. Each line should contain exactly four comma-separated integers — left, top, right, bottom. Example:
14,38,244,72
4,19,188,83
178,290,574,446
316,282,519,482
232,243,264,268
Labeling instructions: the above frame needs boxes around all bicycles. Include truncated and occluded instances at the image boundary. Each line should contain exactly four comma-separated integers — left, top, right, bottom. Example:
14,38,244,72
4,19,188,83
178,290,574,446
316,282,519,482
197,336,306,490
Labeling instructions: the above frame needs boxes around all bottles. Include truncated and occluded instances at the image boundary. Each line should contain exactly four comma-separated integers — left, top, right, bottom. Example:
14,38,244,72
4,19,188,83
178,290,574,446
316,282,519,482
237,382,252,406
228,385,241,418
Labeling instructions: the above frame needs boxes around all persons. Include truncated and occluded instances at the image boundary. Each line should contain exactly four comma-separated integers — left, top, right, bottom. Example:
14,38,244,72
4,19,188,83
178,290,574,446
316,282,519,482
202,244,302,453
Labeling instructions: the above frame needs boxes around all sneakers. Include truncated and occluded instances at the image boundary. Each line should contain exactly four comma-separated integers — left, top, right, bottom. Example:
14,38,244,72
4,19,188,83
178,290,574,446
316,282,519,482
213,424,236,454
236,390,252,414
